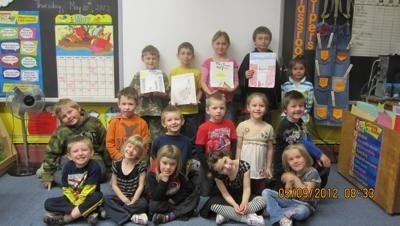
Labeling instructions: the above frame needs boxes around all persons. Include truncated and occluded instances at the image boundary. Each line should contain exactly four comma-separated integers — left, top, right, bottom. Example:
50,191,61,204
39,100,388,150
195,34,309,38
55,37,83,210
201,150,267,226
280,57,314,123
128,45,169,137
275,90,331,187
262,144,322,226
235,92,275,191
42,132,104,226
144,144,199,225
153,105,192,172
202,30,239,124
36,98,106,192
106,87,150,162
99,135,149,226
168,41,202,134
238,25,281,123
195,93,236,159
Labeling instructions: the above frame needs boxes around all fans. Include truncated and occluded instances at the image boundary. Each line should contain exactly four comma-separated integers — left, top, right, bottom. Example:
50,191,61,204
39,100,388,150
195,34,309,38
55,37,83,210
5,81,47,181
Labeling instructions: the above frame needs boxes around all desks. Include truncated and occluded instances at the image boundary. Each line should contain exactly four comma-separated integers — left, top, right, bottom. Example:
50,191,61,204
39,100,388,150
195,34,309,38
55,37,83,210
335,93,400,216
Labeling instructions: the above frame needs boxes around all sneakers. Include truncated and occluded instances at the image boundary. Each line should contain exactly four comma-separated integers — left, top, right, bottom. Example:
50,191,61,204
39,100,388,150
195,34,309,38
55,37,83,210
216,214,226,224
152,213,166,225
43,214,64,226
246,213,265,226
86,212,99,226
130,213,148,225
278,217,293,226
